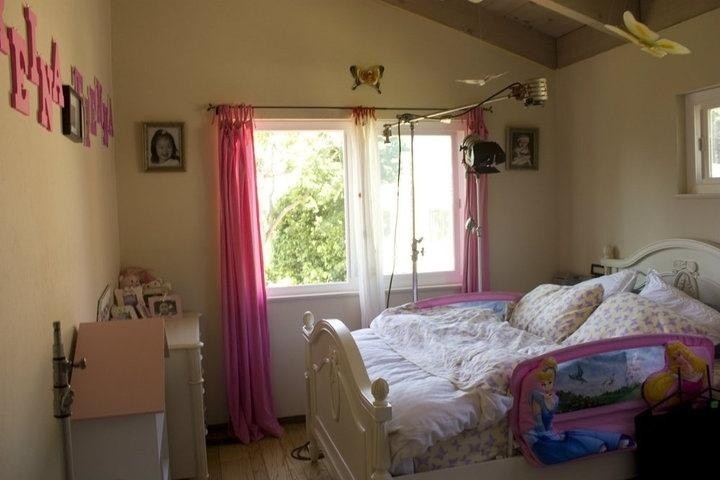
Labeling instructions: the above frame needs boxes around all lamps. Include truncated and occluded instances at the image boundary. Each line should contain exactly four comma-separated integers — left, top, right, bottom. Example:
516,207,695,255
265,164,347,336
382,76,548,302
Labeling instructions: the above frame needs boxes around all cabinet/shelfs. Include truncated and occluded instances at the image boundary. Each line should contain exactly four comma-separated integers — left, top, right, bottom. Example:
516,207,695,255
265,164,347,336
67,310,210,480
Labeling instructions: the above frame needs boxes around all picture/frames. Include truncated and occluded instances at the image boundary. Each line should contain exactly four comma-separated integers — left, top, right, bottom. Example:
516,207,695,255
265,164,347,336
62,83,84,144
139,121,187,172
109,285,182,321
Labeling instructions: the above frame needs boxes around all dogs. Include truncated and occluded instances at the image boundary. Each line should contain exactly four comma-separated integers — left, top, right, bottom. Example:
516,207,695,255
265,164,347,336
159,301,172,318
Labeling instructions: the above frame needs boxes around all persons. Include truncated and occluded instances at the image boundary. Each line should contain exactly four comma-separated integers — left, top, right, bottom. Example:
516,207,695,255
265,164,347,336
158,302,174,317
149,127,179,167
511,132,534,167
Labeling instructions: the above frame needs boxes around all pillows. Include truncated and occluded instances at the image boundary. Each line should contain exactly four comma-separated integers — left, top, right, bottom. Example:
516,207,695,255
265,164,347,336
510,269,720,350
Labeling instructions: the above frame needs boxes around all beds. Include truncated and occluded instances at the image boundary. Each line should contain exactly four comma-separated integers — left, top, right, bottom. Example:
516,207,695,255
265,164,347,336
299,237,719,479
